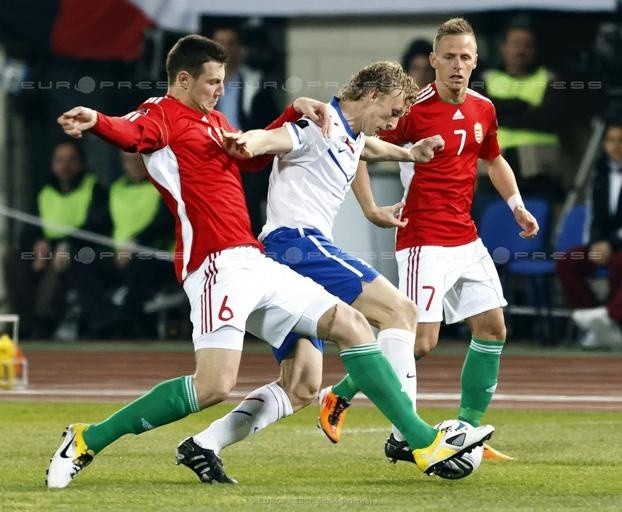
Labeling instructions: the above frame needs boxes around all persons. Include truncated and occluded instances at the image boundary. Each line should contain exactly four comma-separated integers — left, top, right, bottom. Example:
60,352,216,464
319,18,538,463
237,34,276,134
208,27,279,235
398,38,436,85
45,35,494,490
173,60,473,483
75,147,173,342
555,121,622,348
472,25,576,197
4,135,112,343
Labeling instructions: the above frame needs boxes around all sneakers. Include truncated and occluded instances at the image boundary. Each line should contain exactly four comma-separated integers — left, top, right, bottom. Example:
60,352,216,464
175,436,239,486
412,424,495,473
46,423,97,488
385,433,474,479
318,385,352,443
483,442,514,463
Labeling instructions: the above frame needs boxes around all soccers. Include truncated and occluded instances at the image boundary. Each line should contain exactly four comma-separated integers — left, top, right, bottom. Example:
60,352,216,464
432,419,484,479
